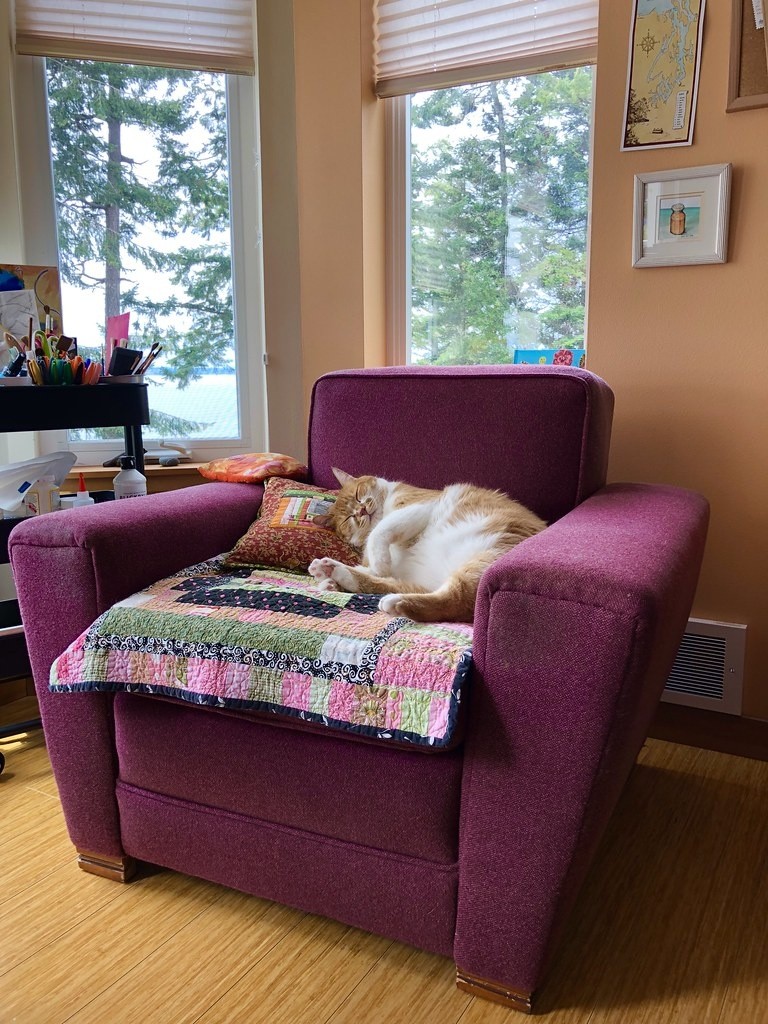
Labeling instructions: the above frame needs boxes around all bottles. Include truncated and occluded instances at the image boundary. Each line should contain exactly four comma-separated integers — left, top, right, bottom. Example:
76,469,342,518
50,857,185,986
73,473,94,507
37,476,61,516
113,456,147,500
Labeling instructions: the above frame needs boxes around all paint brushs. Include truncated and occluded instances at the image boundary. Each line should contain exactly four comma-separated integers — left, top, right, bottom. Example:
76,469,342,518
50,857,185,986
107,338,162,373
41,304,51,334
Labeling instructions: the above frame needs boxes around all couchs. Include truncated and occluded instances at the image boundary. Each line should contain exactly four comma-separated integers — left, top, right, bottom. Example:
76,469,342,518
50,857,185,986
8,366,714,1015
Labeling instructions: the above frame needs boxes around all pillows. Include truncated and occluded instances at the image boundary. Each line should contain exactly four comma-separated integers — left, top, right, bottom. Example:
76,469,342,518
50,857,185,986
220,479,364,570
198,452,308,482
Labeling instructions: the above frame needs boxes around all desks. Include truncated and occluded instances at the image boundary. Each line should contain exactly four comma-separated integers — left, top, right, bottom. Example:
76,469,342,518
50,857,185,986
60,461,215,496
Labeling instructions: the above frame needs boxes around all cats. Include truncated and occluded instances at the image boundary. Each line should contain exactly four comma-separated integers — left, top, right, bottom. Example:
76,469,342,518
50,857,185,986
308,466,549,625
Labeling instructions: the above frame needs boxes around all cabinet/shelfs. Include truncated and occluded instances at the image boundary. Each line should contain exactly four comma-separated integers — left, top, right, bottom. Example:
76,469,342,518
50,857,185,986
0,382,152,773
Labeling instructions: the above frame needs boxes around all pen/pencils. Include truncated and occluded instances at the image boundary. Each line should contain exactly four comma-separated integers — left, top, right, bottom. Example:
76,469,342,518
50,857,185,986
21,328,101,385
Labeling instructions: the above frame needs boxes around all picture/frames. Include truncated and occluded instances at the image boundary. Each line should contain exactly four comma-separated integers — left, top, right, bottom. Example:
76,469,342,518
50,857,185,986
632,163,733,268
724,0,768,114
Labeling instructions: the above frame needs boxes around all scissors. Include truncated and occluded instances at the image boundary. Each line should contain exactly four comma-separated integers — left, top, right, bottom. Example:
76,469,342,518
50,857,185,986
30,328,60,361
